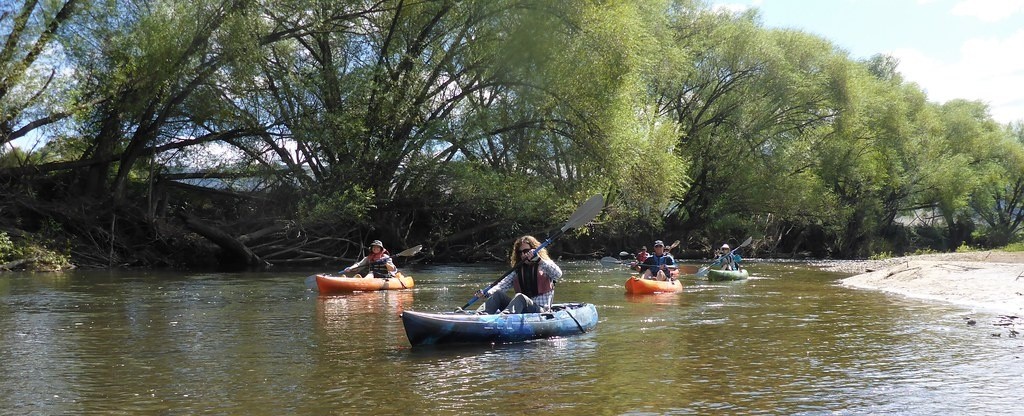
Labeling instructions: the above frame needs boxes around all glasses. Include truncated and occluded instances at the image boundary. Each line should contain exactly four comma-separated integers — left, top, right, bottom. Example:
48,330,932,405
722,249,728,250
517,249,531,256
664,249,670,251
641,249,645,250
655,245,663,248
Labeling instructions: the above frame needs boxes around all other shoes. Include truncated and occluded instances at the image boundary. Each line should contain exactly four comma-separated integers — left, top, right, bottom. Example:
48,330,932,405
354,274,362,278
341,274,346,277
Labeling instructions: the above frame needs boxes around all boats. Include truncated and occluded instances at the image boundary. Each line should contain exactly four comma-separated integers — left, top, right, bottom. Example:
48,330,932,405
631,262,679,277
707,268,748,282
399,302,598,348
316,272,415,294
624,275,682,294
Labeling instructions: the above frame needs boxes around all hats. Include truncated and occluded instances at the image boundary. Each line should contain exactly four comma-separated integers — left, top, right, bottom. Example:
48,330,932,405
641,246,647,251
720,244,730,253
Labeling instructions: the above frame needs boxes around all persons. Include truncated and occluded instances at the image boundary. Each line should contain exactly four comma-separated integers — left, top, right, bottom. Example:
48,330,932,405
344,240,398,278
713,244,741,271
635,240,680,281
454,236,562,314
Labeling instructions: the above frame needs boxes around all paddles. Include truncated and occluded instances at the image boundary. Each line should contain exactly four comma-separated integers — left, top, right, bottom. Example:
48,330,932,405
304,243,423,290
619,251,653,257
601,257,698,274
696,236,752,277
666,239,681,255
457,193,605,310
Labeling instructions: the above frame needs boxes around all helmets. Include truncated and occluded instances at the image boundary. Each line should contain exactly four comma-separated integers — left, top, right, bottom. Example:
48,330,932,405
664,246,670,250
654,240,664,247
371,240,383,250
